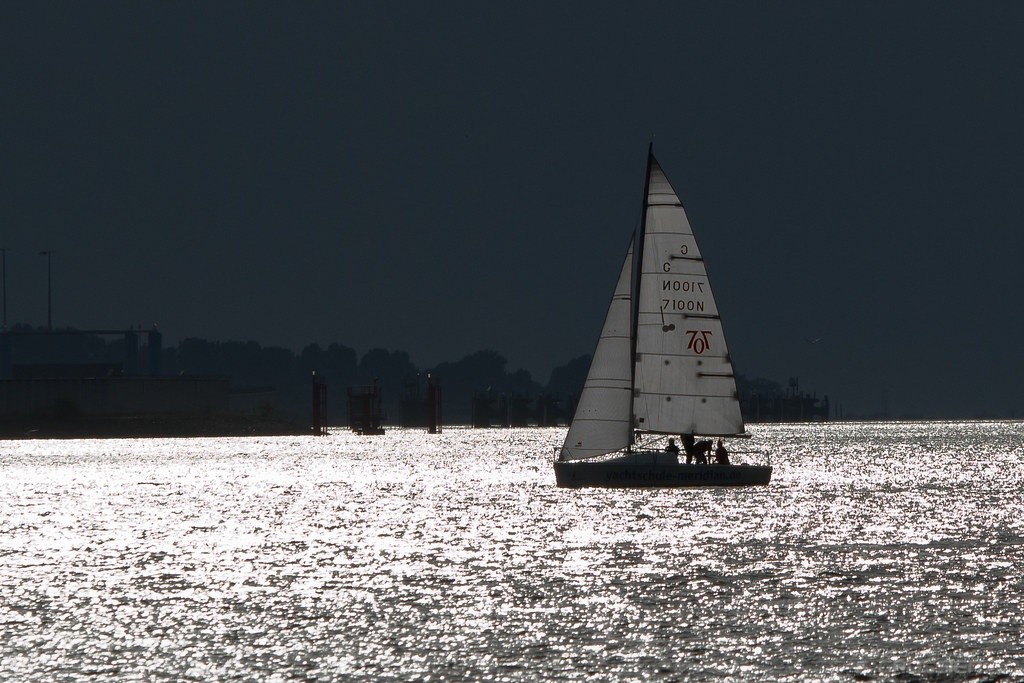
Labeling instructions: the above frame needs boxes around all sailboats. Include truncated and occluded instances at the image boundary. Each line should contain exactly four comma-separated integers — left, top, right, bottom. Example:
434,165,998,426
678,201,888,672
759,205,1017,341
551,137,774,487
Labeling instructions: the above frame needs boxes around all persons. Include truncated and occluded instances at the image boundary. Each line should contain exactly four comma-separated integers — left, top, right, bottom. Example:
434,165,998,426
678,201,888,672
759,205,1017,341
712,441,730,465
665,438,679,459
681,435,694,464
693,440,713,464
